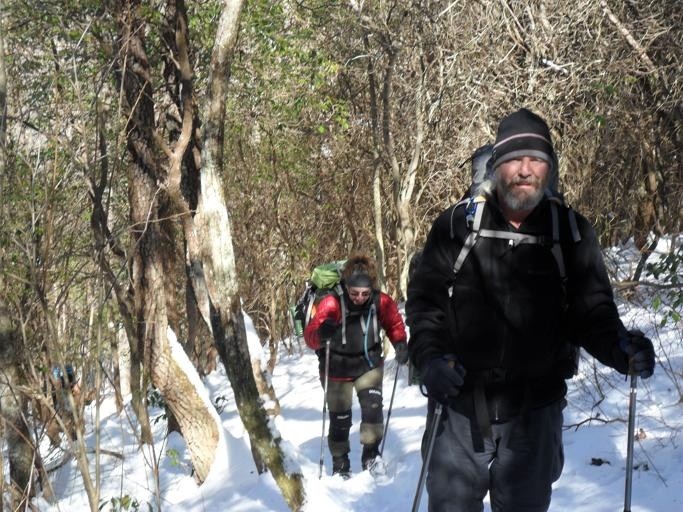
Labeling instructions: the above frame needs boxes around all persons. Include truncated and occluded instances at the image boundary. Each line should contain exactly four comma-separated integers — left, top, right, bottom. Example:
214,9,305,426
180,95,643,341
405,109,655,512
303,255,408,480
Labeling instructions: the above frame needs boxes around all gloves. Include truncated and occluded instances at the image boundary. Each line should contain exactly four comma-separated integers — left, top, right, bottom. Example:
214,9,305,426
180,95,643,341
620,329,655,378
394,341,408,365
320,319,339,337
422,354,467,405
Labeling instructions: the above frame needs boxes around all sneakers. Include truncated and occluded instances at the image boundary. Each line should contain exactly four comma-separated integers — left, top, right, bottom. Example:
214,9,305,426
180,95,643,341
333,454,349,479
361,444,382,470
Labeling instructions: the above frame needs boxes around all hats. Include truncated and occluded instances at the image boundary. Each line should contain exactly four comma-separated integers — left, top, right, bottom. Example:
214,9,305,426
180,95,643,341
491,108,555,172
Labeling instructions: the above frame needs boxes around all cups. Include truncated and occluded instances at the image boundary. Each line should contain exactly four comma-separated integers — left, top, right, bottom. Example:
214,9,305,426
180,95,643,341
290,306,303,337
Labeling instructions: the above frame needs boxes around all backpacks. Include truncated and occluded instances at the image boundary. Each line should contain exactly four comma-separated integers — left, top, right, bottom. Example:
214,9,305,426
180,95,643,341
294,260,380,349
400,193,486,387
